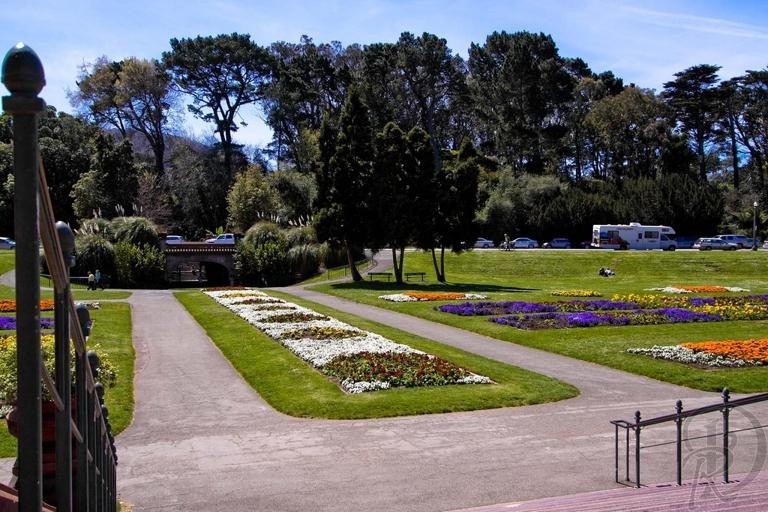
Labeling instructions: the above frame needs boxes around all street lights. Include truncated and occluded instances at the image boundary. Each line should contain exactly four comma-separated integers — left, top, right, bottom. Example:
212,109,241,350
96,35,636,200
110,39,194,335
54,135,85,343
752,201,759,250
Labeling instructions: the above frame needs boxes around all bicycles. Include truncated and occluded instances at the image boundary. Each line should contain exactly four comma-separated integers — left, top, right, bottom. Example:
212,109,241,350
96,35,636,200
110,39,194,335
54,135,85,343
499,241,515,252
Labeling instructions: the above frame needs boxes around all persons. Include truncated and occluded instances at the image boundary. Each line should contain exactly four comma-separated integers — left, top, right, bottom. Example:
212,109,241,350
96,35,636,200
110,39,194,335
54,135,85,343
87,271,95,291
599,267,616,278
94,269,104,290
175,264,201,280
503,233,511,251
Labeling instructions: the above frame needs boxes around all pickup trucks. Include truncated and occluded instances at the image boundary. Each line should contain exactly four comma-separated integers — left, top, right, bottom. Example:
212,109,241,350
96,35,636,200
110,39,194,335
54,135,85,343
205,234,244,245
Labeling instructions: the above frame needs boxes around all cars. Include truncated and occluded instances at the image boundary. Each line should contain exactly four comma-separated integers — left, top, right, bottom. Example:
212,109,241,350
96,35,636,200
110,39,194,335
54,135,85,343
694,235,768,250
580,240,591,249
0,236,16,250
672,236,693,248
498,237,539,249
458,235,495,249
158,235,184,245
542,238,571,248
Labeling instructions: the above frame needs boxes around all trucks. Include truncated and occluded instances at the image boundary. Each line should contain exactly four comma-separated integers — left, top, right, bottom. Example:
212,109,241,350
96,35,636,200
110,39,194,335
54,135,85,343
591,224,678,251
